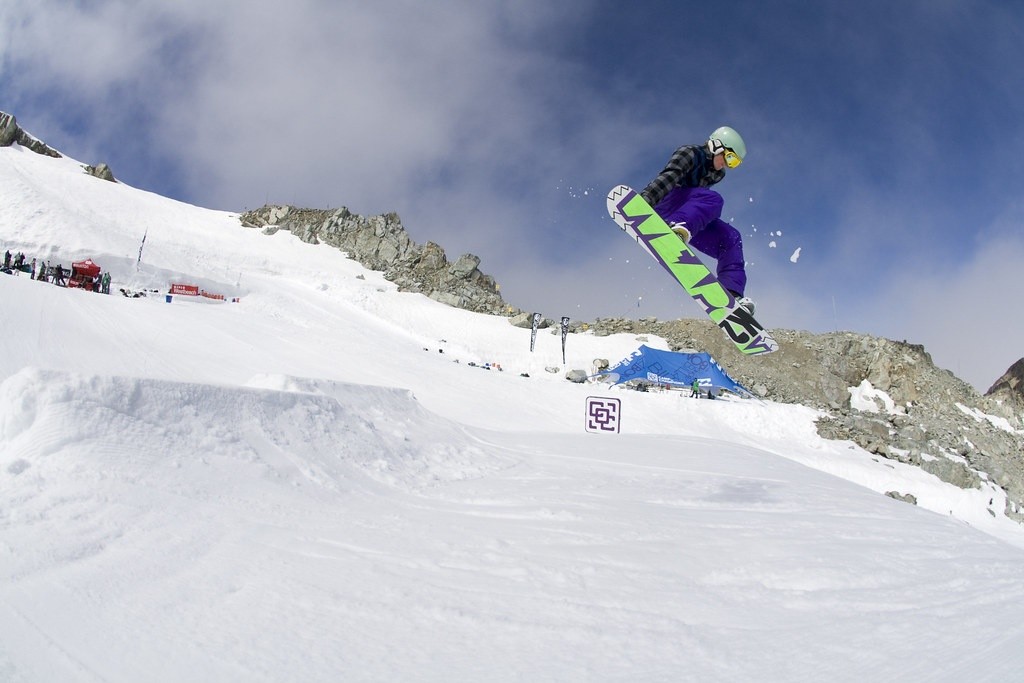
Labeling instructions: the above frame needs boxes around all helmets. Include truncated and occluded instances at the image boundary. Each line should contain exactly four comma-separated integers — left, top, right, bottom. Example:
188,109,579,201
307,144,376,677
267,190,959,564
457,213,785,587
710,125,747,161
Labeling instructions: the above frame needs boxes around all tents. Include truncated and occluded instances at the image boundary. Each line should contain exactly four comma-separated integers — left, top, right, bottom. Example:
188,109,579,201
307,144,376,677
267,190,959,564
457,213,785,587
70,258,101,292
572,343,768,406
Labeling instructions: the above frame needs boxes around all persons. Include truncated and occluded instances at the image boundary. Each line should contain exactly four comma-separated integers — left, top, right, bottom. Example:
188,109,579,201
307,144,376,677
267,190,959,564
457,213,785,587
56,264,65,286
51,264,57,285
19,253,25,270
38,261,45,280
31,258,36,280
81,270,112,294
689,378,700,398
45,260,50,274
13,251,21,269
4,250,11,268
640,126,757,315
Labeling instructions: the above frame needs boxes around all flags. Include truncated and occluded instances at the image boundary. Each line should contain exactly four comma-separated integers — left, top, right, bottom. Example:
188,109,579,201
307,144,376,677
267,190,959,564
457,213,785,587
529,312,542,351
561,317,570,364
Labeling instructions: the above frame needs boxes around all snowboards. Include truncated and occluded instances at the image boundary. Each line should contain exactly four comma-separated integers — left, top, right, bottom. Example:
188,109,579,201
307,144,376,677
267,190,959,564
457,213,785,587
604,185,781,355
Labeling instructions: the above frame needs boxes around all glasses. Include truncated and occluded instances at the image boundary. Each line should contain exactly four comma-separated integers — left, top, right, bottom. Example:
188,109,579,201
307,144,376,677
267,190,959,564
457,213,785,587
722,148,741,168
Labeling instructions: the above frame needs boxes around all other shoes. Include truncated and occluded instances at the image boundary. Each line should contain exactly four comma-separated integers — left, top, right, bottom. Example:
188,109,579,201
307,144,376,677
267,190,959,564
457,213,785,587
734,295,757,317
669,222,692,244
